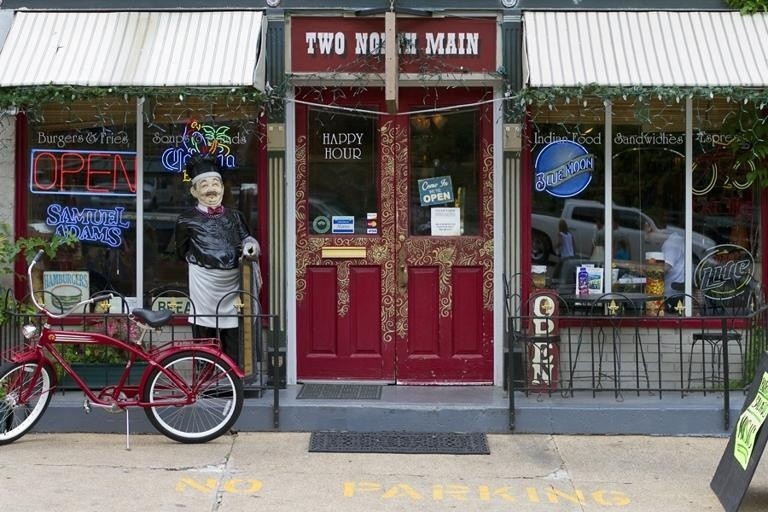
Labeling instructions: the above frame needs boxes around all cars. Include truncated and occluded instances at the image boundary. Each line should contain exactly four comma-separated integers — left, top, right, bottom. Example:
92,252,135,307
29,207,194,292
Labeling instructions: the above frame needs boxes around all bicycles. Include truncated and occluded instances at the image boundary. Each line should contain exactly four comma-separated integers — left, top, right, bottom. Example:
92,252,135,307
0,249,247,453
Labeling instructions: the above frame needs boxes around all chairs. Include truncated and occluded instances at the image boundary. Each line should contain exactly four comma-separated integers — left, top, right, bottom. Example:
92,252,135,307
504,273,569,400
683,272,747,397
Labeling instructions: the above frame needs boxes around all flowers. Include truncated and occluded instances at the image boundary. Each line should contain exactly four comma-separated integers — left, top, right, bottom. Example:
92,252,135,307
49,316,161,384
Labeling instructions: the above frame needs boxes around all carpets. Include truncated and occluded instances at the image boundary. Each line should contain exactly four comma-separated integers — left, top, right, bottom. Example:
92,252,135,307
307,431,492,455
296,382,383,399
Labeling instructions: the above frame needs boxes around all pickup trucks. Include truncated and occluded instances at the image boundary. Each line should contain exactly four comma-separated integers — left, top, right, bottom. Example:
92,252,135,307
531,197,718,278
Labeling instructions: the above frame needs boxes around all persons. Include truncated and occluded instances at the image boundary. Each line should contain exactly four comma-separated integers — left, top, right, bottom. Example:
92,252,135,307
175,158,259,380
642,225,685,298
557,219,575,256
591,220,631,262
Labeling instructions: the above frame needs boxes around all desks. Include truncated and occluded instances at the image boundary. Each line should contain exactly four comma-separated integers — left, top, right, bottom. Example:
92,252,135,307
560,291,666,400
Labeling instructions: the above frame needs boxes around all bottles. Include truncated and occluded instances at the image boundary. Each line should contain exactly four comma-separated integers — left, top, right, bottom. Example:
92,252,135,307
577,267,590,297
